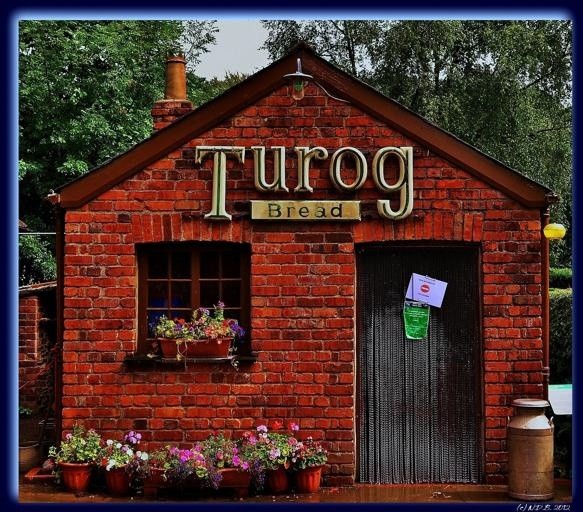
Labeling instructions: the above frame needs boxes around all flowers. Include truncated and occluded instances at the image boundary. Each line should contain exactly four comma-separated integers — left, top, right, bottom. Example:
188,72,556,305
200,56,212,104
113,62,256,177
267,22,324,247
142,298,246,373
44,422,329,499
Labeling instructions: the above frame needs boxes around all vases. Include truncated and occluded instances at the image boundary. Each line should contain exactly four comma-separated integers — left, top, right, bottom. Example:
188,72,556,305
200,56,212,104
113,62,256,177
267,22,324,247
156,335,233,360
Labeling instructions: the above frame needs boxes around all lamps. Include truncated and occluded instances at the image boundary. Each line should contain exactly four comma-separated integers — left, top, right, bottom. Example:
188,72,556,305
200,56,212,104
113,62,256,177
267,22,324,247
284,56,316,102
540,191,566,243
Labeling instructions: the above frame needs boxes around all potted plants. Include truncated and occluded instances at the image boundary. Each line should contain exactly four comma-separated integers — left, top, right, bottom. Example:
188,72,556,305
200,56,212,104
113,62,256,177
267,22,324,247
18,406,40,473
